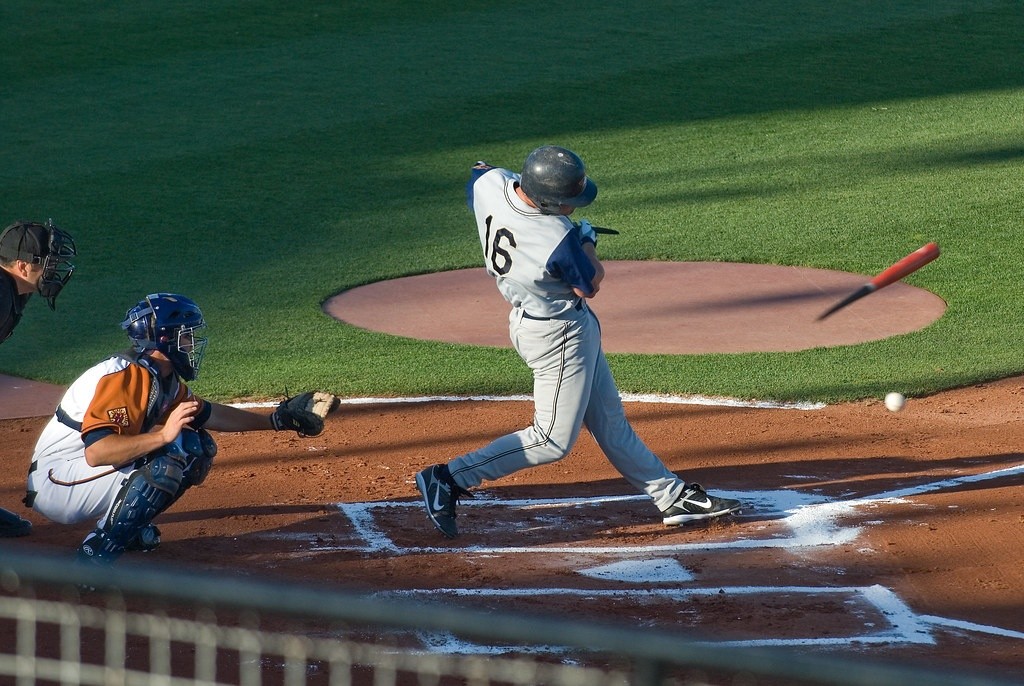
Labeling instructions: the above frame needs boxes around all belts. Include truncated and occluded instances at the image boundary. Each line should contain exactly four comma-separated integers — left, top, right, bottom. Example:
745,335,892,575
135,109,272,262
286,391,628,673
523,298,582,320
29,461,37,474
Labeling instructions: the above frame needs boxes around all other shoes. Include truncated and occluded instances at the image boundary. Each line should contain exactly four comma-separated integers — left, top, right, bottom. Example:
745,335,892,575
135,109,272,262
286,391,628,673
0,508,32,538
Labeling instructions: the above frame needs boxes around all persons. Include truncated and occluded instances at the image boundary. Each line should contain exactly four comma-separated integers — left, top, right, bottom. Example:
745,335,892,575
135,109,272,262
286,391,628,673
414,145,741,537
25,292,338,593
0,218,76,348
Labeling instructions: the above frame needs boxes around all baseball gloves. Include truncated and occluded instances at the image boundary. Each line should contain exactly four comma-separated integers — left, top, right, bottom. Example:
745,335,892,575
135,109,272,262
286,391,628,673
276,384,341,438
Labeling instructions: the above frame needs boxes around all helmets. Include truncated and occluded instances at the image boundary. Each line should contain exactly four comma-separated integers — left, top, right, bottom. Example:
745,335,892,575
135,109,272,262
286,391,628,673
125,294,205,341
521,146,598,214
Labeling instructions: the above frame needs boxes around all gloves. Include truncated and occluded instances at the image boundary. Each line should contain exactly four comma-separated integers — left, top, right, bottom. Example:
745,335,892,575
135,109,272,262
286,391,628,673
573,220,597,247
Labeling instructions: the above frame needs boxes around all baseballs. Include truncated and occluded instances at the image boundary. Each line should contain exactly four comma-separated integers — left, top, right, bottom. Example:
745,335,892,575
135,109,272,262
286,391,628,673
885,391,905,412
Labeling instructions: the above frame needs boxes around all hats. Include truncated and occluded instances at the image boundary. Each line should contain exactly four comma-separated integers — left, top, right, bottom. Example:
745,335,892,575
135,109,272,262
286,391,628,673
0,221,71,264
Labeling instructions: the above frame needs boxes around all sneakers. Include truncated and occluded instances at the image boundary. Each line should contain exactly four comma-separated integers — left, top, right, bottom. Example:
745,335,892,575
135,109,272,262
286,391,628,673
416,464,474,539
663,483,741,525
77,528,125,567
97,518,161,552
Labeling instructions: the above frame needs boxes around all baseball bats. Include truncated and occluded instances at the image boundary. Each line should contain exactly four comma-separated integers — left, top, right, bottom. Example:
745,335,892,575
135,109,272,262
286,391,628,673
810,239,943,325
